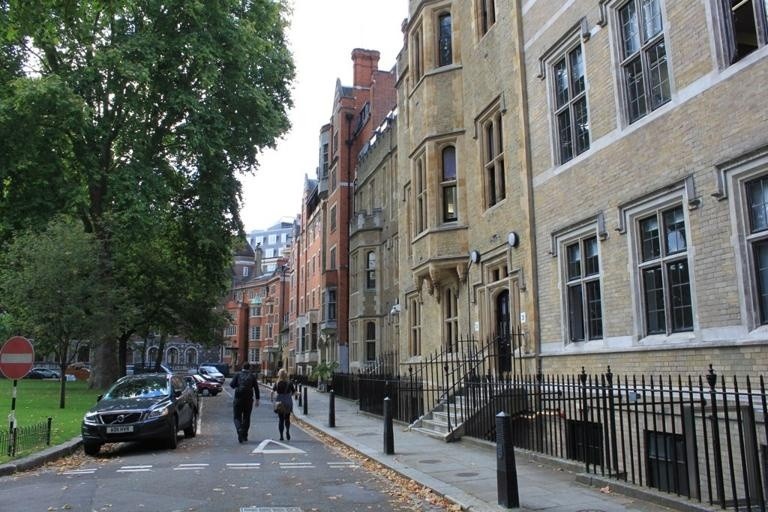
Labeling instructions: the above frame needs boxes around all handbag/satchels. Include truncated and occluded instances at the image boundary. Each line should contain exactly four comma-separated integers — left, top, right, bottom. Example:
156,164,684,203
274,401,285,415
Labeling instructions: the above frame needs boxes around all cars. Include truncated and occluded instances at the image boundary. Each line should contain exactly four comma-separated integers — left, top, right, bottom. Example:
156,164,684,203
24,368,60,378
122,359,229,397
65,361,91,380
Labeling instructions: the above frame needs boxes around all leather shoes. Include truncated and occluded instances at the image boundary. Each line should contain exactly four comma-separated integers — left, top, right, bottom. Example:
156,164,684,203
237,428,244,443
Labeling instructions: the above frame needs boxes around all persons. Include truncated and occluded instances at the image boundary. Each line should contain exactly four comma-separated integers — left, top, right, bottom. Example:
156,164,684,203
268,368,296,442
227,363,259,444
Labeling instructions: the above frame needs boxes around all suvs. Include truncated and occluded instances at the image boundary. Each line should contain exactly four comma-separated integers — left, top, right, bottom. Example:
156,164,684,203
79,373,199,454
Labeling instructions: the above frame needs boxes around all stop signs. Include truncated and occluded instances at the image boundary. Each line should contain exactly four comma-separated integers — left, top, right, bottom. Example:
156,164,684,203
0,336,35,379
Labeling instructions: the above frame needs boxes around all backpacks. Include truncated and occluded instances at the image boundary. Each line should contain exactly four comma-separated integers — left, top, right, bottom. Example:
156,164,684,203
235,372,252,400
